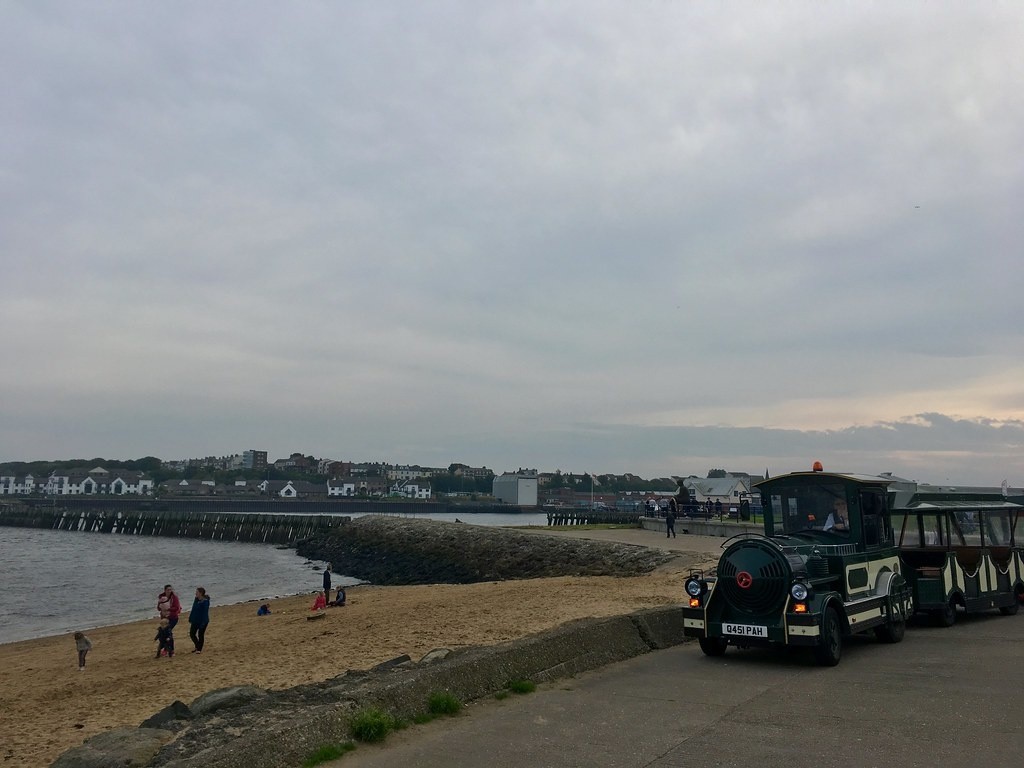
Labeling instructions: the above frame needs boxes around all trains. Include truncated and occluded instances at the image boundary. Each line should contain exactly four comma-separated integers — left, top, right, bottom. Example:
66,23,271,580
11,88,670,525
683,460,1024,667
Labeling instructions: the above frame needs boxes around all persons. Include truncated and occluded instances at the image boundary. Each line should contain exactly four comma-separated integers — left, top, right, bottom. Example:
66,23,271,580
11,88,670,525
705,497,722,520
153,585,182,659
188,587,210,654
822,495,850,533
256,604,272,616
74,632,93,671
311,564,346,611
646,496,669,518
666,511,676,538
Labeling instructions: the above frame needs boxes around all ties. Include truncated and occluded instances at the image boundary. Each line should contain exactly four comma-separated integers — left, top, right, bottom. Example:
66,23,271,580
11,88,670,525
841,516,844,524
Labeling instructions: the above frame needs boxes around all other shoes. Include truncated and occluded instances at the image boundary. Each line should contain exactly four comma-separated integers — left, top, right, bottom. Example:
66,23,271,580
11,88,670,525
196,650,200,654
76,666,85,670
191,647,197,652
